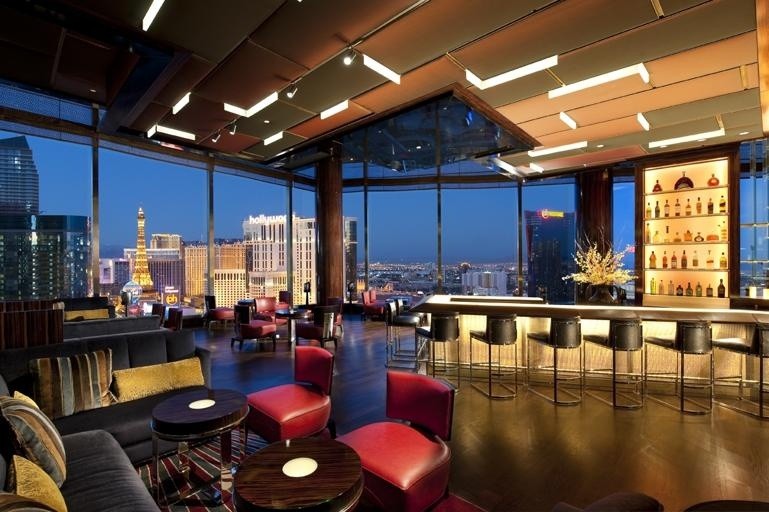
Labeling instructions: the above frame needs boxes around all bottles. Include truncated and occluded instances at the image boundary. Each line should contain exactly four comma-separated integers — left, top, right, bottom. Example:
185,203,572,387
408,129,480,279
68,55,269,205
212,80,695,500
644,171,728,298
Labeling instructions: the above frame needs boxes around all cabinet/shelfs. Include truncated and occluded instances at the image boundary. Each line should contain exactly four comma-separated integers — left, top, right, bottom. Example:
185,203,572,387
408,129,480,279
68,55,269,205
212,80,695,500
634,140,739,311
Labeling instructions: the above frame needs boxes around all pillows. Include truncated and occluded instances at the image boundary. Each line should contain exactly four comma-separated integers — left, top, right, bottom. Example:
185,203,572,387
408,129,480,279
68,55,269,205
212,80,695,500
0,346,205,512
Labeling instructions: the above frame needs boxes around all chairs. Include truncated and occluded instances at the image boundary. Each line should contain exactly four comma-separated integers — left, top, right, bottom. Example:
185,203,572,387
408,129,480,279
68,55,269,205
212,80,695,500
360,290,385,321
526,320,580,406
152,304,183,331
415,312,461,396
385,298,415,369
583,320,645,410
644,321,711,415
335,370,455,512
712,323,768,422
240,346,334,460
209,291,343,352
469,315,517,400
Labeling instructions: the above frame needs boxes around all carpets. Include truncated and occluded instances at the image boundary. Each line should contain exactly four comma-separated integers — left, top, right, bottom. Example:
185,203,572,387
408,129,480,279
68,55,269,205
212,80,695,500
132,423,489,512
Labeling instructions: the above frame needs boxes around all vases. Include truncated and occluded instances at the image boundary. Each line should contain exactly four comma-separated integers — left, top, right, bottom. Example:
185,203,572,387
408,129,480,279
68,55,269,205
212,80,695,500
588,283,613,306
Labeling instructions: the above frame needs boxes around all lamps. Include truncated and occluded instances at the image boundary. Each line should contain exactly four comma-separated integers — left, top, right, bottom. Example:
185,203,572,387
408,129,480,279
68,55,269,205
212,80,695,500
212,122,237,144
286,85,298,99
343,45,356,66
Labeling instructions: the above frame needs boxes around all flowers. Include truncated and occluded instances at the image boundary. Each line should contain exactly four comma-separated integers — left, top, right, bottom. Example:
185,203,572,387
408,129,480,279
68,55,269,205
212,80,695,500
560,225,639,285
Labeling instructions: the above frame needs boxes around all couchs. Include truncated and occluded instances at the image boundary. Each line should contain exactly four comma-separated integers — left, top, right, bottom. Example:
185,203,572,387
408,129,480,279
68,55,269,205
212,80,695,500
53,297,114,322
61,315,160,340
0,329,212,468
0,377,161,511
